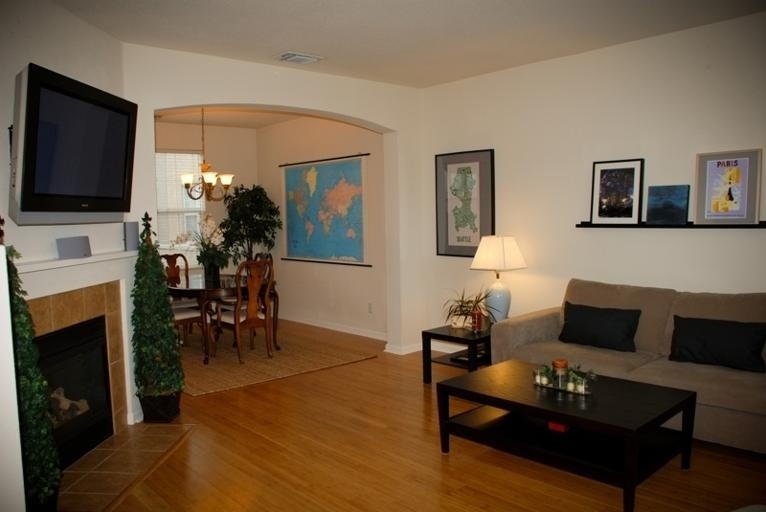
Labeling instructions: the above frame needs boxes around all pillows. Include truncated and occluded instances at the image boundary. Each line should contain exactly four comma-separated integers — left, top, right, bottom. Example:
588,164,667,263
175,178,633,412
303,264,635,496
669,313,766,372
559,300,642,353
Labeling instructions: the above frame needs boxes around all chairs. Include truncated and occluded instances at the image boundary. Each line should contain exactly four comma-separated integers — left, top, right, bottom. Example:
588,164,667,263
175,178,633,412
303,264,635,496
211,259,276,366
170,309,205,348
158,252,199,309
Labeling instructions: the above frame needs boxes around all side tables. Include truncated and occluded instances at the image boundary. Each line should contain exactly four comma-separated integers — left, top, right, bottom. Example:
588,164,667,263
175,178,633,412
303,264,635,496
421,322,491,383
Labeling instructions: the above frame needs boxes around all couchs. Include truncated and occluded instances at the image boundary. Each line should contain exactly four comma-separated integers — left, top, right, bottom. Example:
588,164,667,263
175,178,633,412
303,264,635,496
490,276,766,458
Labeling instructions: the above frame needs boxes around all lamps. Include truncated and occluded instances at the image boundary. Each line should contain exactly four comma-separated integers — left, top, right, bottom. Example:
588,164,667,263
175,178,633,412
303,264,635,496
180,108,235,202
469,236,528,324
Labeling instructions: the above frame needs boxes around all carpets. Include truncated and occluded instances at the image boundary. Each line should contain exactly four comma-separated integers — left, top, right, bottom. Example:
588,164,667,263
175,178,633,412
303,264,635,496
181,323,378,402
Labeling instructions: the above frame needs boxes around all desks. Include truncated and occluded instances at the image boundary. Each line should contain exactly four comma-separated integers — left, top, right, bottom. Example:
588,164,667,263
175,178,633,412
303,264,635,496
165,274,281,365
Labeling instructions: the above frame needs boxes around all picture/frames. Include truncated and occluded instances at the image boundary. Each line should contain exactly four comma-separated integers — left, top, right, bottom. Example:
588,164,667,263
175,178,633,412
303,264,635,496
433,149,496,258
692,147,763,224
590,158,646,227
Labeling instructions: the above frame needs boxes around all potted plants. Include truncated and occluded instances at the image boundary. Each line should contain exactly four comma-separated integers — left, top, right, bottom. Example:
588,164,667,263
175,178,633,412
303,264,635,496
130,212,184,422
441,287,500,329
7,245,62,511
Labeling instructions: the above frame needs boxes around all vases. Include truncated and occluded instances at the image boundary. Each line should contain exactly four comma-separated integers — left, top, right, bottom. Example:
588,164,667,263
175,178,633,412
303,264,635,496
204,262,221,277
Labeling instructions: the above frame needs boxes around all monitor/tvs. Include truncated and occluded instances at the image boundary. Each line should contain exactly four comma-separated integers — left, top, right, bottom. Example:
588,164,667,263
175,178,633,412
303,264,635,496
8,60,138,227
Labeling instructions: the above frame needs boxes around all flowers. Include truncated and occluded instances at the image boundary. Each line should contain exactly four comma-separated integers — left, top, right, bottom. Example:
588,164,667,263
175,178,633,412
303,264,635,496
169,213,230,261
